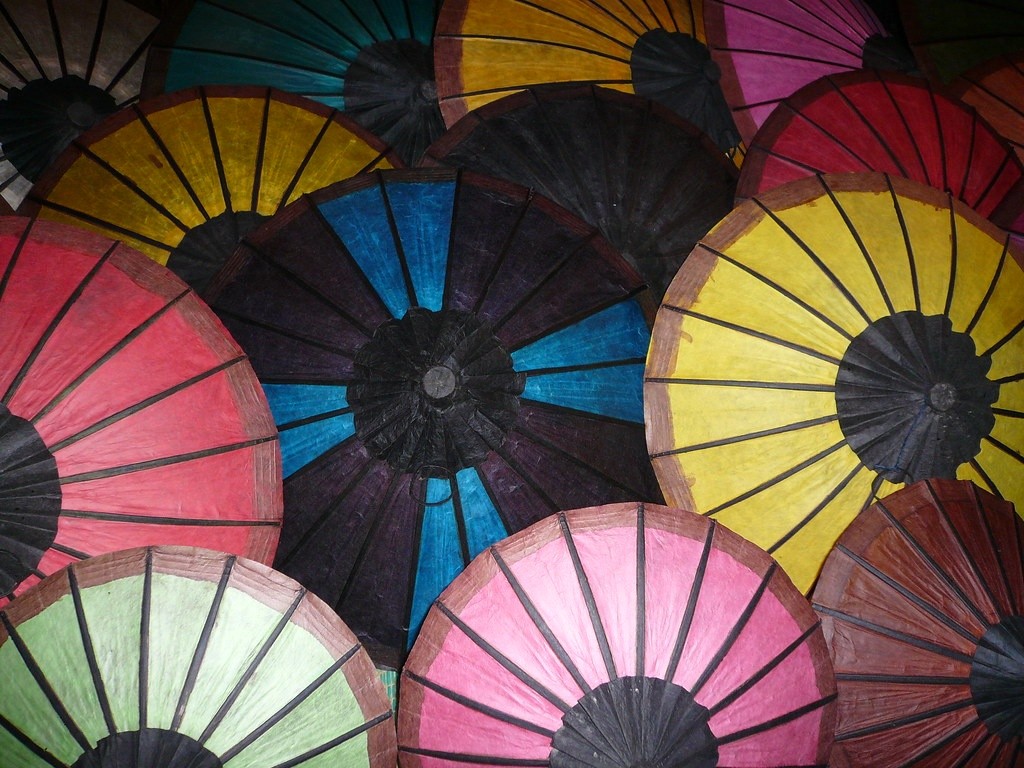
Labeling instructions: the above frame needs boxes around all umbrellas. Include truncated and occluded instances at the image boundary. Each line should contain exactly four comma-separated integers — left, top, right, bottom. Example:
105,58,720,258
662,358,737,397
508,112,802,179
0,0,1024,667
2,547,398,768
811,476,1024,768
399,500,842,768
